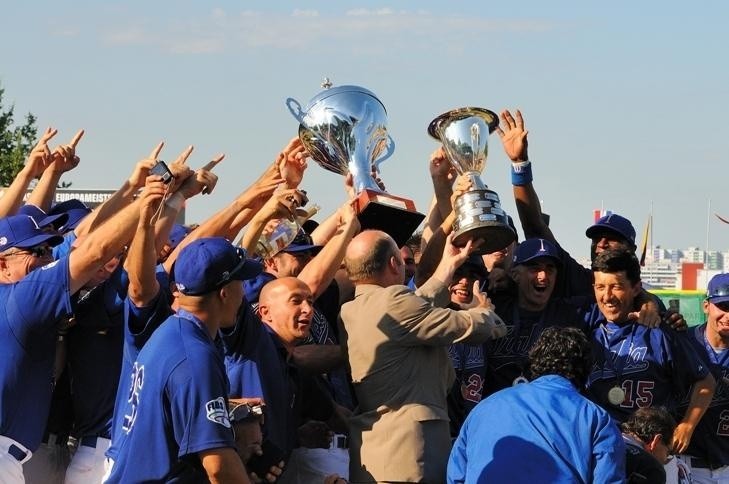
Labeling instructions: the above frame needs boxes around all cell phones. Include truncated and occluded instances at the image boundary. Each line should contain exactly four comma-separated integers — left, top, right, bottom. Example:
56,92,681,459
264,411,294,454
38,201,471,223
247,441,285,482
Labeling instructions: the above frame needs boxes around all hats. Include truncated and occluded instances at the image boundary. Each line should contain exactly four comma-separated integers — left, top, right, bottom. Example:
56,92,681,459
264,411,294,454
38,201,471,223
174,237,264,297
17,203,63,228
706,274,729,304
586,213,635,243
169,224,188,248
271,222,325,255
0,214,65,252
49,198,92,235
513,238,560,265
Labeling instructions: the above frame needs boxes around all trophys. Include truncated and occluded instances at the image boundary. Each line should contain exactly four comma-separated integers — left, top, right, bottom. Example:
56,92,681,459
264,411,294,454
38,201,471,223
284,75,427,249
428,106,518,255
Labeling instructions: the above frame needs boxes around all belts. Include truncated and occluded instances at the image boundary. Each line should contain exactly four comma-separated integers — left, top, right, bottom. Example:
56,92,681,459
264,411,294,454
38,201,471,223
335,438,350,448
9,444,26,462
81,436,97,448
677,456,724,468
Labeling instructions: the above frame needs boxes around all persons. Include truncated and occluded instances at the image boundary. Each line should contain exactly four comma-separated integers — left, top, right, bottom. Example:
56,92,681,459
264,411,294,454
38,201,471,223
680,273,729,484
446,325,628,484
153,136,570,484
103,235,285,484
495,112,716,484
2,123,226,484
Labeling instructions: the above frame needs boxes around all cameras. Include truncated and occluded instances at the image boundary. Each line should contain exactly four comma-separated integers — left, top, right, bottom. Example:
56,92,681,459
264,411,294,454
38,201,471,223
148,161,172,184
287,189,309,208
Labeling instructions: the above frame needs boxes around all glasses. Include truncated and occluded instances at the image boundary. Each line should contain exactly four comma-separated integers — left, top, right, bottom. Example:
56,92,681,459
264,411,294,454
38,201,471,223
215,247,248,287
290,235,315,247
229,404,265,427
